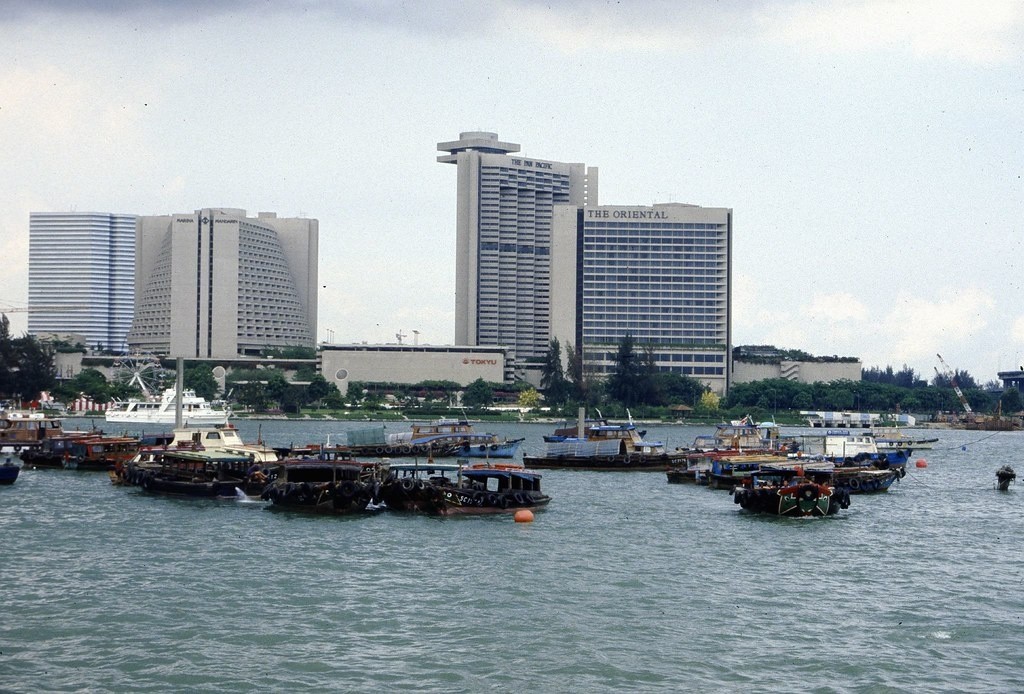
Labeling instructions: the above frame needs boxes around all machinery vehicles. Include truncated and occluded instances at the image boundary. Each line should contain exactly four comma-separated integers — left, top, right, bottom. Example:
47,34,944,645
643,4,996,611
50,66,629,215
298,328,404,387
937,353,992,423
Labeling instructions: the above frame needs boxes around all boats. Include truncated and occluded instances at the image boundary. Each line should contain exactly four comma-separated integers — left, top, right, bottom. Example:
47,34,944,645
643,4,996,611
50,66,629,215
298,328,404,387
0,354,940,524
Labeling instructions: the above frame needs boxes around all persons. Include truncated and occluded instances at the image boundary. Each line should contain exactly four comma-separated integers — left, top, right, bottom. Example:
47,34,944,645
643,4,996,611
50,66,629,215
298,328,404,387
775,437,799,453
759,480,792,489
